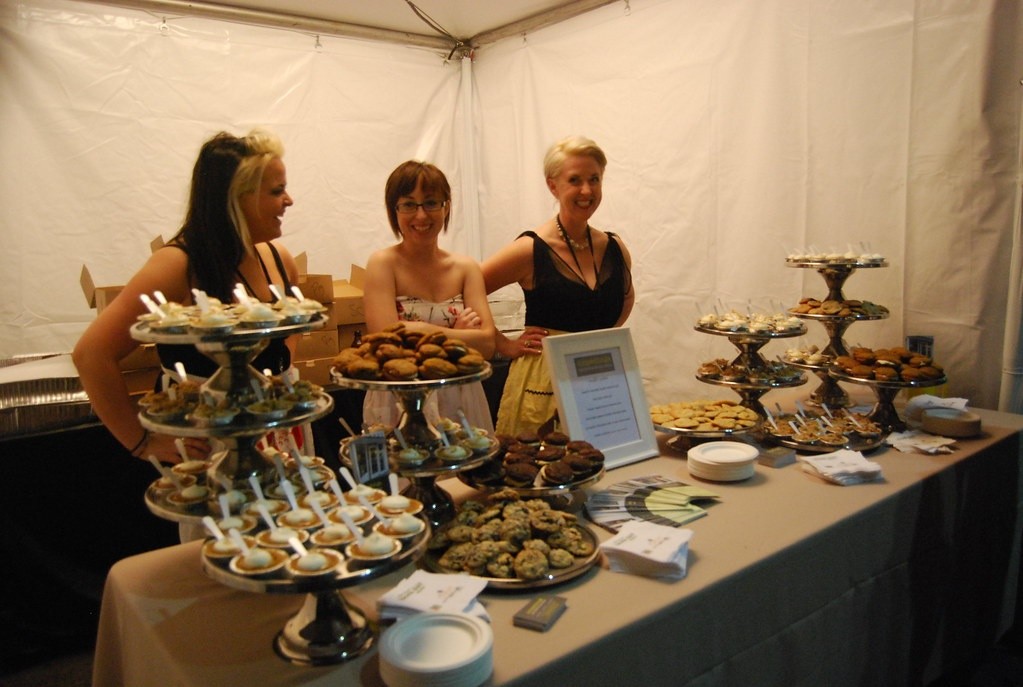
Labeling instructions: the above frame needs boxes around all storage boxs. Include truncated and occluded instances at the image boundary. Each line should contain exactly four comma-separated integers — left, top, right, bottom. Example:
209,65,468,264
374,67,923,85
84,237,368,391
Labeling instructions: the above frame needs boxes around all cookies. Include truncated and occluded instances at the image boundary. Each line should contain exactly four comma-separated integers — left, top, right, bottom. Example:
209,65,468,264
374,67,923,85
789,297,886,316
427,489,593,580
648,400,759,432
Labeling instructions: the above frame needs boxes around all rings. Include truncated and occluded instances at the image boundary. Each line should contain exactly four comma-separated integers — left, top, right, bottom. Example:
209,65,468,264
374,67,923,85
525,341,532,347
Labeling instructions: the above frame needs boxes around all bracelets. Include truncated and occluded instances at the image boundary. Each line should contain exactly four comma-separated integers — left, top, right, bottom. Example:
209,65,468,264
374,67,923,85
130,430,150,458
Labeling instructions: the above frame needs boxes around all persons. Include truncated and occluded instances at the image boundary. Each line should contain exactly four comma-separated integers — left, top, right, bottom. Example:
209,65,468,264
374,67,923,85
364,159,495,437
72,131,313,543
480,135,635,445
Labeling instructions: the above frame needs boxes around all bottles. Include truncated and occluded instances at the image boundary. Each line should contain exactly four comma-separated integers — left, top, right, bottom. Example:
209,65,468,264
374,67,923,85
351,329,362,348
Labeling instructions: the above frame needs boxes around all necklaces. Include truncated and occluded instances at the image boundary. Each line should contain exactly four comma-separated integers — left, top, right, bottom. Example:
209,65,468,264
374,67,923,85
555,216,589,250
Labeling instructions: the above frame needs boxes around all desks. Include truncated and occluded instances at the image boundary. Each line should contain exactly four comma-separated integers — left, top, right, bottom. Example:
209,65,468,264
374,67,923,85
0,359,515,677
92,397,1023,687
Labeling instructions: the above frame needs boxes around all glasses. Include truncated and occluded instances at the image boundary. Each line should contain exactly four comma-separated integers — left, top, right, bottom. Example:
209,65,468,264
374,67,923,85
394,199,447,214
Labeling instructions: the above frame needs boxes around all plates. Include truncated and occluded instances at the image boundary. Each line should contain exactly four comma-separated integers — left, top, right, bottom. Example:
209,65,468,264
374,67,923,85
377,610,494,687
686,440,759,482
922,409,982,438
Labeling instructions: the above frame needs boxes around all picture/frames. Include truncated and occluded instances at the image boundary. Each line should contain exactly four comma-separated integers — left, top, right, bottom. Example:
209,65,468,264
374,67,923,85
541,325,661,471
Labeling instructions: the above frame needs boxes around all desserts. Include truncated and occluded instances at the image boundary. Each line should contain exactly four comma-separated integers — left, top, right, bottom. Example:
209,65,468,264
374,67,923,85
695,296,804,334
784,341,945,382
333,321,485,383
696,350,806,383
137,282,427,582
763,400,882,445
786,241,885,263
470,432,604,488
337,408,492,470
